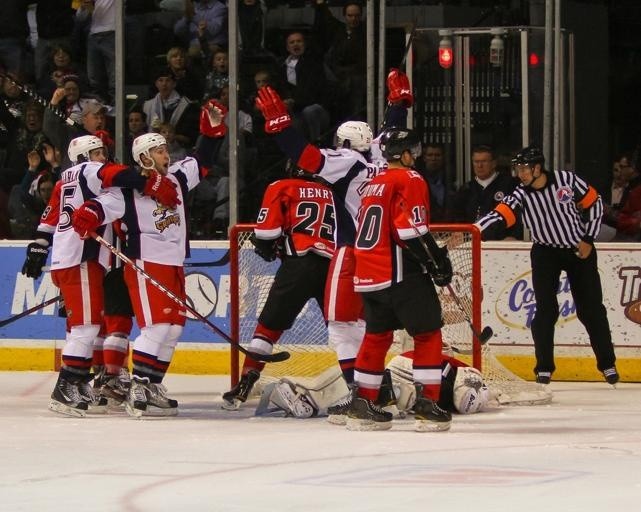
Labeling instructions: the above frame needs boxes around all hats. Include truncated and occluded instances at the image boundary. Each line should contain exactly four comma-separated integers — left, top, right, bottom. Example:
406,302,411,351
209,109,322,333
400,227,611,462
80,98,107,117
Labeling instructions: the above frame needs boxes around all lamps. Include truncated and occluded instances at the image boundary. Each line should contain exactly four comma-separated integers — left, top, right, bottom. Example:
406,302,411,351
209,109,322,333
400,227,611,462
437,27,455,71
489,28,508,68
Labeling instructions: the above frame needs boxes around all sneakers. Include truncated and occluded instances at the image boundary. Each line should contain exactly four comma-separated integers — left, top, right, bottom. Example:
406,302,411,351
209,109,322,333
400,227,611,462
603,365,618,384
536,372,551,384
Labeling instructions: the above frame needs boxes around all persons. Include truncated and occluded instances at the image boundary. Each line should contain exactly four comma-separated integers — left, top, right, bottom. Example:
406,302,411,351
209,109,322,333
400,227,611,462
255,350,501,418
419,144,524,241
91,129,134,402
222,140,373,402
445,143,619,384
253,69,415,415
603,148,638,241
346,126,451,423
20,135,181,409
1,2,379,241
71,97,227,412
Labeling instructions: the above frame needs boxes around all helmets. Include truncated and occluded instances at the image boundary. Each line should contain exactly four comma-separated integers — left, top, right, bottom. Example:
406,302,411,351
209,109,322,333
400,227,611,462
332,121,373,153
66,135,108,165
510,146,544,172
452,366,488,414
284,158,314,179
131,132,166,164
379,126,422,161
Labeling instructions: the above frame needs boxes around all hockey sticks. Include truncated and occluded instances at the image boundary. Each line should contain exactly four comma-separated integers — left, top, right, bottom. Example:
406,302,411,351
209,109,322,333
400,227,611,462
89,232,290,363
408,218,492,344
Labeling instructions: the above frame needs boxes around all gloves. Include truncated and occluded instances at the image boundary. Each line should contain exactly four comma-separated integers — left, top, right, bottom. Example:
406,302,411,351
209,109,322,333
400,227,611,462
386,68,413,108
426,255,452,286
254,246,276,262
254,85,291,134
71,201,104,240
21,242,48,280
142,169,180,211
199,98,227,138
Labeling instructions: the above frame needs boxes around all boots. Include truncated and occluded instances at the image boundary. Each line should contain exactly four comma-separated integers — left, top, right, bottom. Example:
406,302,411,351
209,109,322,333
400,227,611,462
222,370,259,404
50,365,177,411
346,381,392,422
412,381,451,422
326,369,397,415
